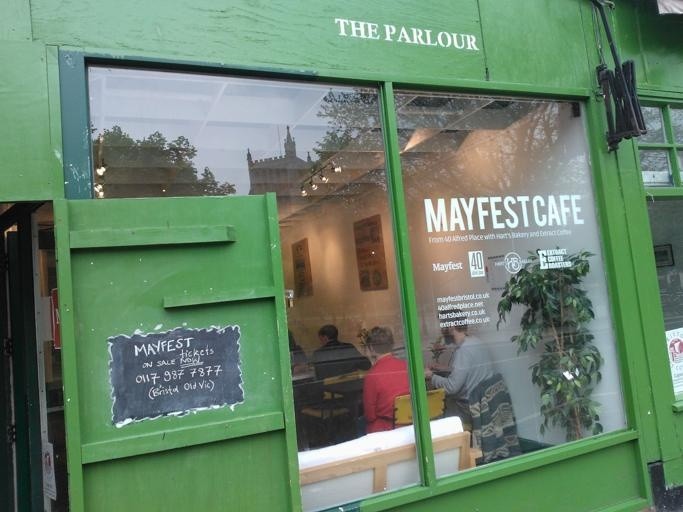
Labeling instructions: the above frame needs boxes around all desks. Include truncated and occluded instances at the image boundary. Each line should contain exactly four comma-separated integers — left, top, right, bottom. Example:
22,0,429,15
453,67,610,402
292,372,317,399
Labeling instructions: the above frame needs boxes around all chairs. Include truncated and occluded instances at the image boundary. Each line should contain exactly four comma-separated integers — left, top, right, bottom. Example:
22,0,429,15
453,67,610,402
302,367,514,463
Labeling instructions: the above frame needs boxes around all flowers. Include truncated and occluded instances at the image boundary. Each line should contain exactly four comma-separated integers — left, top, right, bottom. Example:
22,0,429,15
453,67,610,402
426,337,446,364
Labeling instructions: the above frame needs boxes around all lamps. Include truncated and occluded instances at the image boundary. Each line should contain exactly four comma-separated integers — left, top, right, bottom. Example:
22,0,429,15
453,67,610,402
299,161,342,197
94,165,108,199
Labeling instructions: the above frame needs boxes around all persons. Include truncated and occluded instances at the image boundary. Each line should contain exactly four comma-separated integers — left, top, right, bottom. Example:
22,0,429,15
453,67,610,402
288,334,308,376
357,327,408,438
310,325,370,410
422,309,496,424
